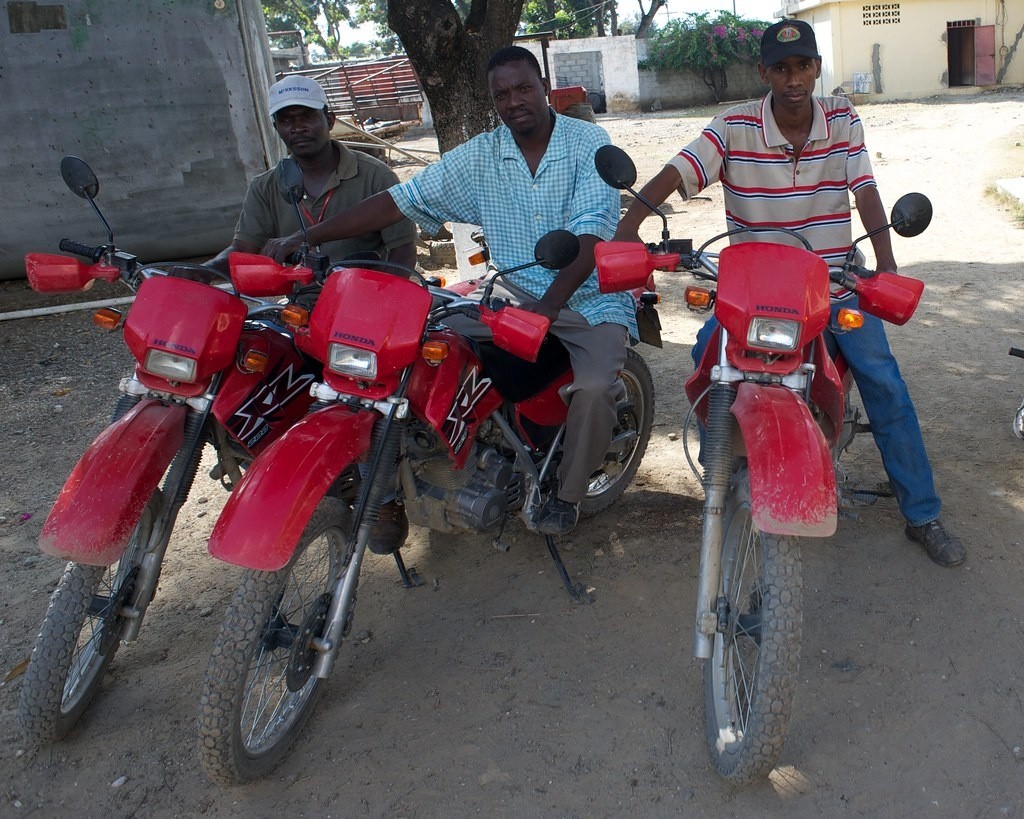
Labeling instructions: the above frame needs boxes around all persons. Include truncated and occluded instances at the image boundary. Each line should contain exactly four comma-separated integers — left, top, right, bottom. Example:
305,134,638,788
608,19,969,568
261,47,641,535
167,74,416,556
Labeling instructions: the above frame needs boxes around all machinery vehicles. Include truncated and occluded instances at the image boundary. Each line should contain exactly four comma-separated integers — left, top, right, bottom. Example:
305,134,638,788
511,31,596,126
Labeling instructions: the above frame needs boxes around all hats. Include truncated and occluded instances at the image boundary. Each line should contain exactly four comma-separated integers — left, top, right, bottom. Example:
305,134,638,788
760,19,821,67
266,73,327,118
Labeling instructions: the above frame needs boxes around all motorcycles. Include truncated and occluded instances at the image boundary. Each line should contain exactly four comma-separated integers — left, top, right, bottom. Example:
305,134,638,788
198,159,694,789
595,144,932,788
19,155,490,746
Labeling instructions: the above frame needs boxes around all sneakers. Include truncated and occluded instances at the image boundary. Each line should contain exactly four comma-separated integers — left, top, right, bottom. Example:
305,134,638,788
538,494,580,534
904,519,966,567
364,502,409,555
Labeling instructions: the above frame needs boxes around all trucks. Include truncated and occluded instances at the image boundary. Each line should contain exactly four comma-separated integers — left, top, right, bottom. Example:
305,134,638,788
265,30,425,157
